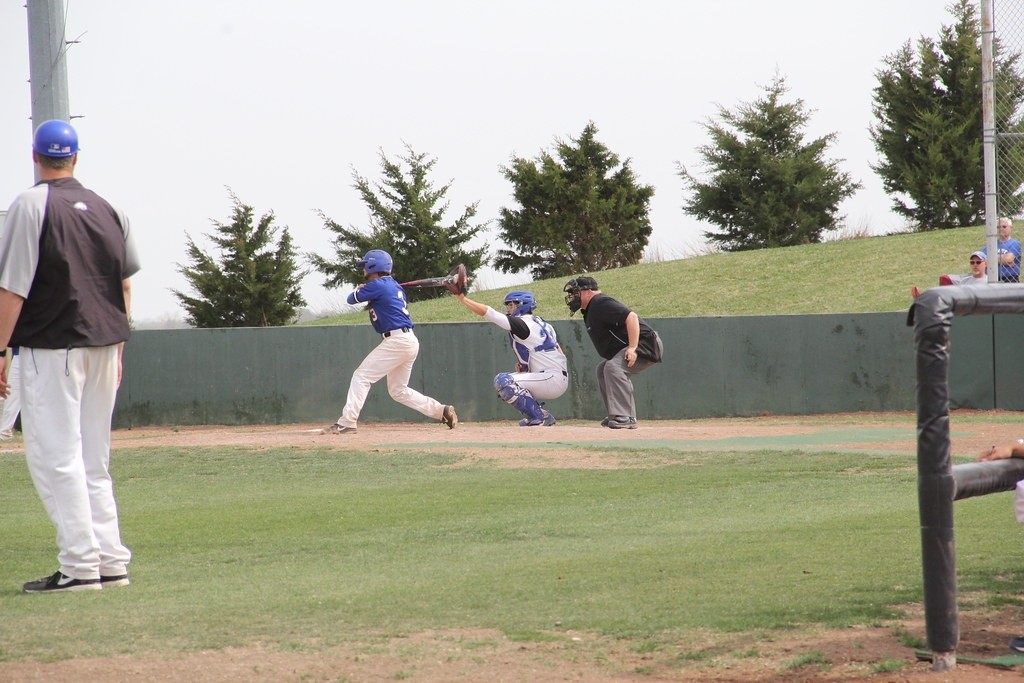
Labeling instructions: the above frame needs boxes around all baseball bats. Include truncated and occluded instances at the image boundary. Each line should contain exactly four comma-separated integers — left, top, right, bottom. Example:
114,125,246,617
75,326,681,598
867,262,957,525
399,275,474,286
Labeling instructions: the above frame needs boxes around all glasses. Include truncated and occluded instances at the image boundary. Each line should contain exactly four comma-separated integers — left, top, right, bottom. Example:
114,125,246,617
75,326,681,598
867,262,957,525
997,225,1007,229
970,260,984,265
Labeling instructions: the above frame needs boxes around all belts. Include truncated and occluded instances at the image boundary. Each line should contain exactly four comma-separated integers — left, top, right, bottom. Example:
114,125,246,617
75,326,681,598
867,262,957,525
383,327,409,336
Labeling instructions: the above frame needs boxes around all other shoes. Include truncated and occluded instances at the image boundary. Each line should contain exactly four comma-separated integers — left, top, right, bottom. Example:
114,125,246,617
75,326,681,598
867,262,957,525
911,284,920,298
600,416,638,429
519,413,556,426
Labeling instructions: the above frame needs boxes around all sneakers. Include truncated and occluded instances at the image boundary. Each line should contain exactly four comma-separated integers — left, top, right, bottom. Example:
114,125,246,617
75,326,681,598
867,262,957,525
100,574,130,587
322,421,358,434
442,403,458,429
23,568,102,594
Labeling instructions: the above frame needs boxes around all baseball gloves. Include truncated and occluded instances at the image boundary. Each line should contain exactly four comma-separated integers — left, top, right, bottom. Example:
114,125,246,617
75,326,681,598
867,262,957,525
443,263,469,296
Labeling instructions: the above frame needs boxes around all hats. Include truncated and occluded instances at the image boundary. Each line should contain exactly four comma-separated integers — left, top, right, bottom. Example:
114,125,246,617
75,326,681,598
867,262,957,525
539,370,567,378
576,276,598,289
969,251,986,260
33,119,79,157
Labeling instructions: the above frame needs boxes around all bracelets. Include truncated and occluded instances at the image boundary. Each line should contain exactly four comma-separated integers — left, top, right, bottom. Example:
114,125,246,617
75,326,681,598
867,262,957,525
1015,438,1024,446
0,350,6,356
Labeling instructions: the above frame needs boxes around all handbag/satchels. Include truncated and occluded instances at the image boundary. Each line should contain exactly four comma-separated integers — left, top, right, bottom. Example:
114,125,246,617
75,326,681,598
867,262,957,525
635,334,663,364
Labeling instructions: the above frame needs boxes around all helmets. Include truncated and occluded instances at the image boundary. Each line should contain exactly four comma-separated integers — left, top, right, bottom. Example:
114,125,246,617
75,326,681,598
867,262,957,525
356,249,393,275
504,290,537,318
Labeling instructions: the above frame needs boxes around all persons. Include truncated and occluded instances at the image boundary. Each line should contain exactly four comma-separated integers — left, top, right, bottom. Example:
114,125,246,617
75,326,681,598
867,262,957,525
976,438,1024,528
0,120,141,592
455,292,569,426
563,276,664,429
323,250,458,435
960,252,988,285
982,217,1020,282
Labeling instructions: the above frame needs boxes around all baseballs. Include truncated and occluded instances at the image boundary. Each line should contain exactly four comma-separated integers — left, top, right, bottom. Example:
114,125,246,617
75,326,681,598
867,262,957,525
452,273,459,284
